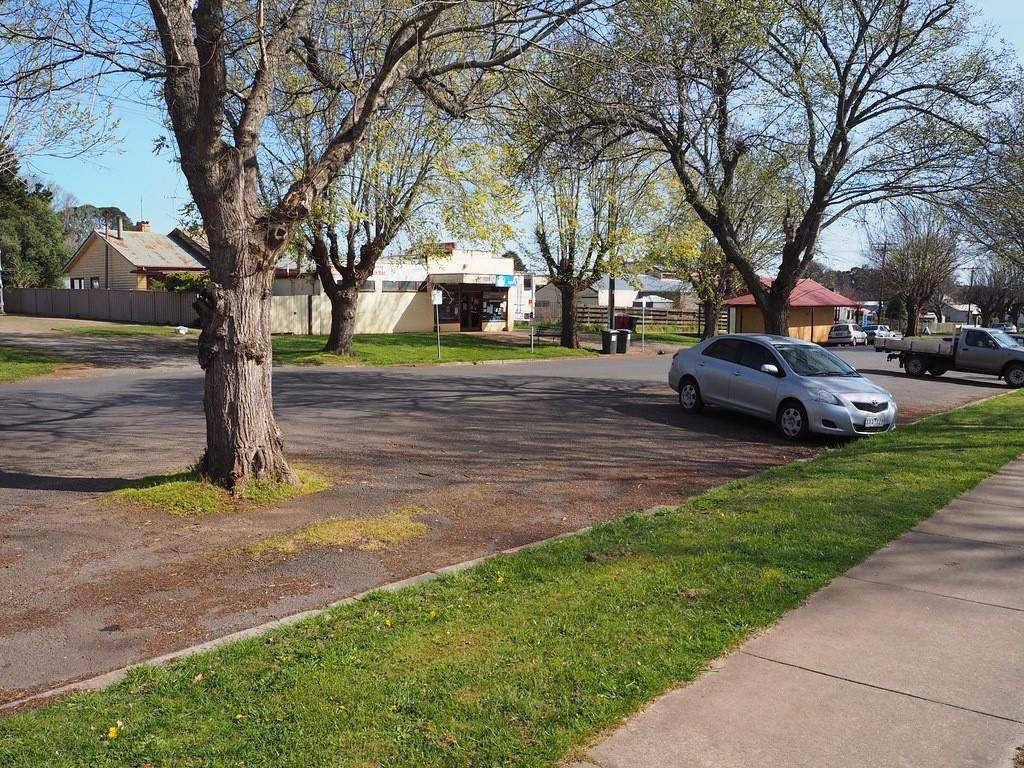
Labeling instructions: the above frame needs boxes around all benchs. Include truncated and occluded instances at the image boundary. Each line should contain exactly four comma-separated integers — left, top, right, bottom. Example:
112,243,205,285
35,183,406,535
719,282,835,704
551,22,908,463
529,325,562,346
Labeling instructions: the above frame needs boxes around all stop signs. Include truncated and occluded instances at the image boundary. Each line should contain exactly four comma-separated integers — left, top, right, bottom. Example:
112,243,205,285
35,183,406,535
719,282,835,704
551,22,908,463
920,307,928,316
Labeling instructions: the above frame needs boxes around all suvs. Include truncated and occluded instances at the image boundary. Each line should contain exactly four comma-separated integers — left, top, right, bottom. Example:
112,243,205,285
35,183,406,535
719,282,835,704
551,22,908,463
994,322,1017,333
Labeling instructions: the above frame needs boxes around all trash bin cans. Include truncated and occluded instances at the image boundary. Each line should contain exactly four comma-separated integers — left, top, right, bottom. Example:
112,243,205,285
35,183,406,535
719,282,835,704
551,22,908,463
600,329,620,354
615,316,624,331
617,329,633,354
624,317,640,333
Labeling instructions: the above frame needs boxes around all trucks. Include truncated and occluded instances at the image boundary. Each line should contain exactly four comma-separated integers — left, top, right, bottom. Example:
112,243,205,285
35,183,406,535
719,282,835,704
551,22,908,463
874,325,1024,388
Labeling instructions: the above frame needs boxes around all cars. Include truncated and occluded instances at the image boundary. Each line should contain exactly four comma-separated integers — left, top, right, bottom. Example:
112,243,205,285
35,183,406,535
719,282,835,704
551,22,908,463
668,331,898,438
828,324,867,347
862,325,896,343
